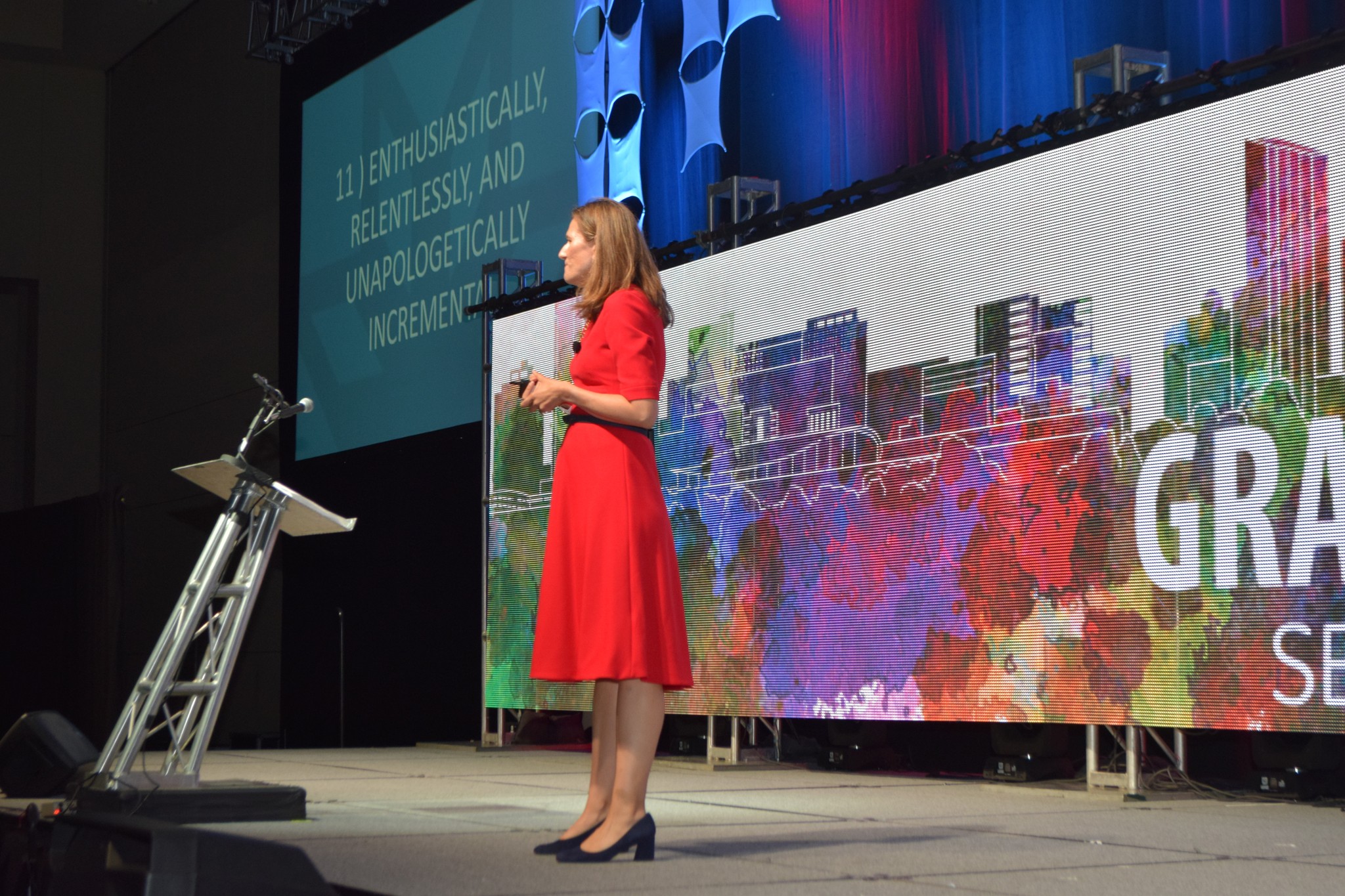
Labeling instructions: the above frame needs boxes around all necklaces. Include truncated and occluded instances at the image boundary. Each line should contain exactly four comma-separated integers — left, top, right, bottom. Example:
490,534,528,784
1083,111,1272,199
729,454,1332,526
572,320,592,354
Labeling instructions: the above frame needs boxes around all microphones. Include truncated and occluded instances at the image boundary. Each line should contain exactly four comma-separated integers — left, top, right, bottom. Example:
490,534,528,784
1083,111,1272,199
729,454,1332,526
572,341,581,353
264,398,314,425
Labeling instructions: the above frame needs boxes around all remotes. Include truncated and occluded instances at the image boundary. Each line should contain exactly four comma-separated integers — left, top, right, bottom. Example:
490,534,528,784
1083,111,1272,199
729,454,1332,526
510,379,530,398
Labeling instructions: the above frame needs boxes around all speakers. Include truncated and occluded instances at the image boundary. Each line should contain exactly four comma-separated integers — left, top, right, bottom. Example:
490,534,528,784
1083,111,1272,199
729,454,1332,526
0,710,99,799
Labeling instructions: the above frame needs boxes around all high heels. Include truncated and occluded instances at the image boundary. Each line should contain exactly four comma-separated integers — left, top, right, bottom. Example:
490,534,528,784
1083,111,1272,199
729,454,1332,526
556,813,656,864
533,818,629,855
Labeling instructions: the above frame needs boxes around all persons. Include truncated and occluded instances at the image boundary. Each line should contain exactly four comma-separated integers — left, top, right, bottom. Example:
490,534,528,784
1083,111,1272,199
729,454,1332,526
517,198,693,865
